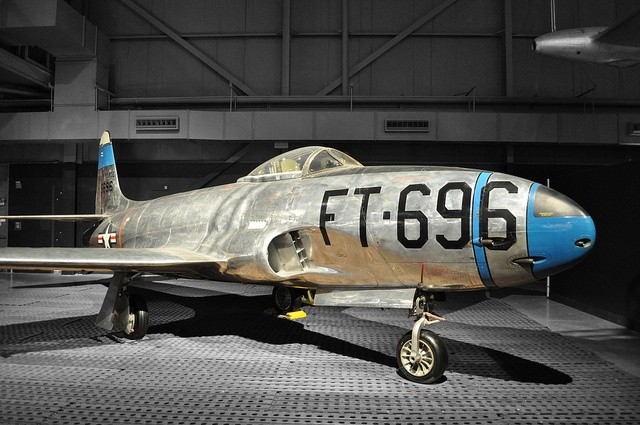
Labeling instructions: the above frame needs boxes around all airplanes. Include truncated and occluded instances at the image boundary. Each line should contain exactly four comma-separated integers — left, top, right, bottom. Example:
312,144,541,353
1,128,597,385
